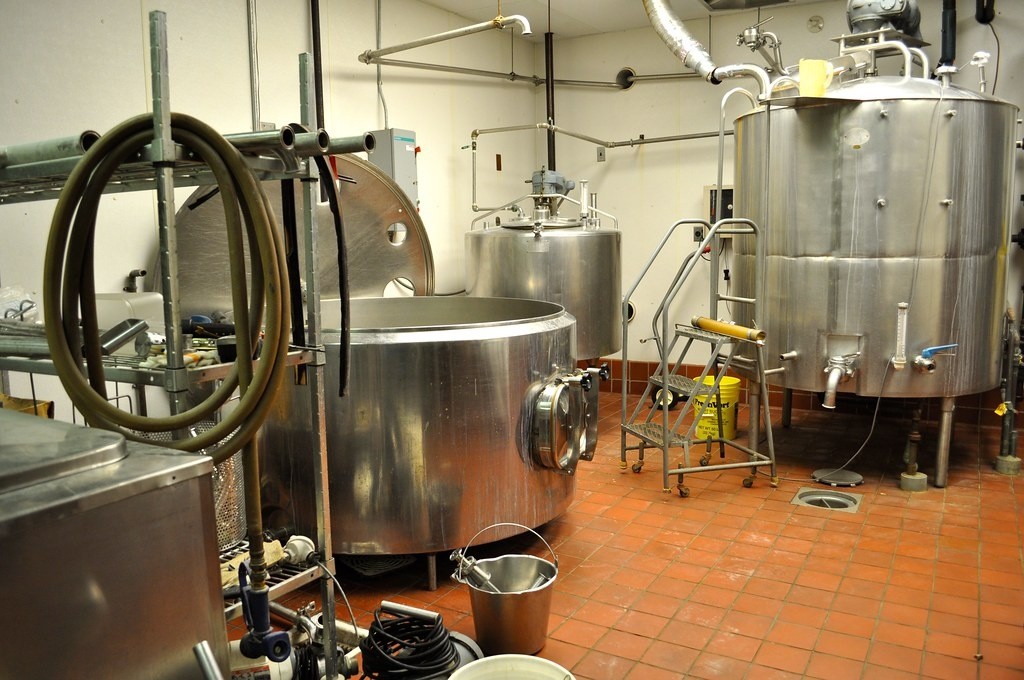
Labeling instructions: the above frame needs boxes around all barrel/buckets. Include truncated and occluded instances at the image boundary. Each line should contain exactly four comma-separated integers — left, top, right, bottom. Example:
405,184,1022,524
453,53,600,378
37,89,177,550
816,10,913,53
449,523,559,655
691,375,742,440
446,654,577,680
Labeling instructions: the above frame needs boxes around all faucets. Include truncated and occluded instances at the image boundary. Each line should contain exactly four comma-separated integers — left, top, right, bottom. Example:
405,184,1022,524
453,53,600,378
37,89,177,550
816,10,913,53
915,343,959,374
820,356,851,410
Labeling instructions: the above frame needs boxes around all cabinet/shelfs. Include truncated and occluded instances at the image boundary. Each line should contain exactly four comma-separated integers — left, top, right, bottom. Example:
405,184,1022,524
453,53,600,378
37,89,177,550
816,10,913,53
0,9,339,680
